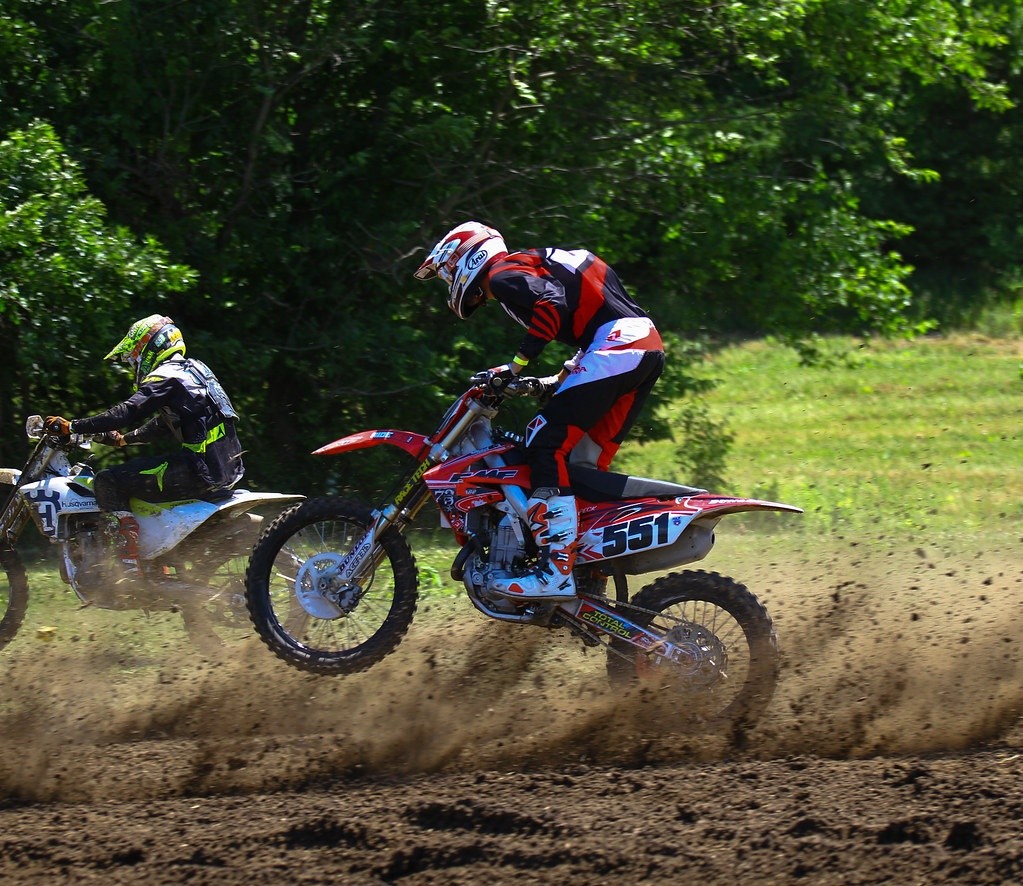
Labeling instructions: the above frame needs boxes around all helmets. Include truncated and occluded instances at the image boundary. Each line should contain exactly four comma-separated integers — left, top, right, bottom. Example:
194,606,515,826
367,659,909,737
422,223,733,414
415,222,508,321
103,314,186,390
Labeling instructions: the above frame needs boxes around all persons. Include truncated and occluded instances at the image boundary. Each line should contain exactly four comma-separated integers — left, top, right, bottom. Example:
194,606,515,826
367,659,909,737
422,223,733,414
412,220,664,601
42,314,246,592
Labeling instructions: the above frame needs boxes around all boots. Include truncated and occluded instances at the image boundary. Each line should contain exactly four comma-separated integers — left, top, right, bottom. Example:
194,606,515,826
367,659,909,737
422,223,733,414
75,512,146,593
494,489,578,601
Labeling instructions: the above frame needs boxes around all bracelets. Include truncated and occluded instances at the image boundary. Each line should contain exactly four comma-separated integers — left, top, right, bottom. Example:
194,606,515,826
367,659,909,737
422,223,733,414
513,355,529,366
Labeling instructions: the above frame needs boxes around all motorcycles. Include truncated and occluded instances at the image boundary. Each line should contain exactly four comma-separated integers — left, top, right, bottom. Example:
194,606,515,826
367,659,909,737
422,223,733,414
246,370,807,732
0,411,308,658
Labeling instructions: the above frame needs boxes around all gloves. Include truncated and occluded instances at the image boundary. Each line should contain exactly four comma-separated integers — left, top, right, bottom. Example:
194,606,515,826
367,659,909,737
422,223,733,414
487,364,517,392
536,375,560,397
93,430,126,447
45,416,74,438
